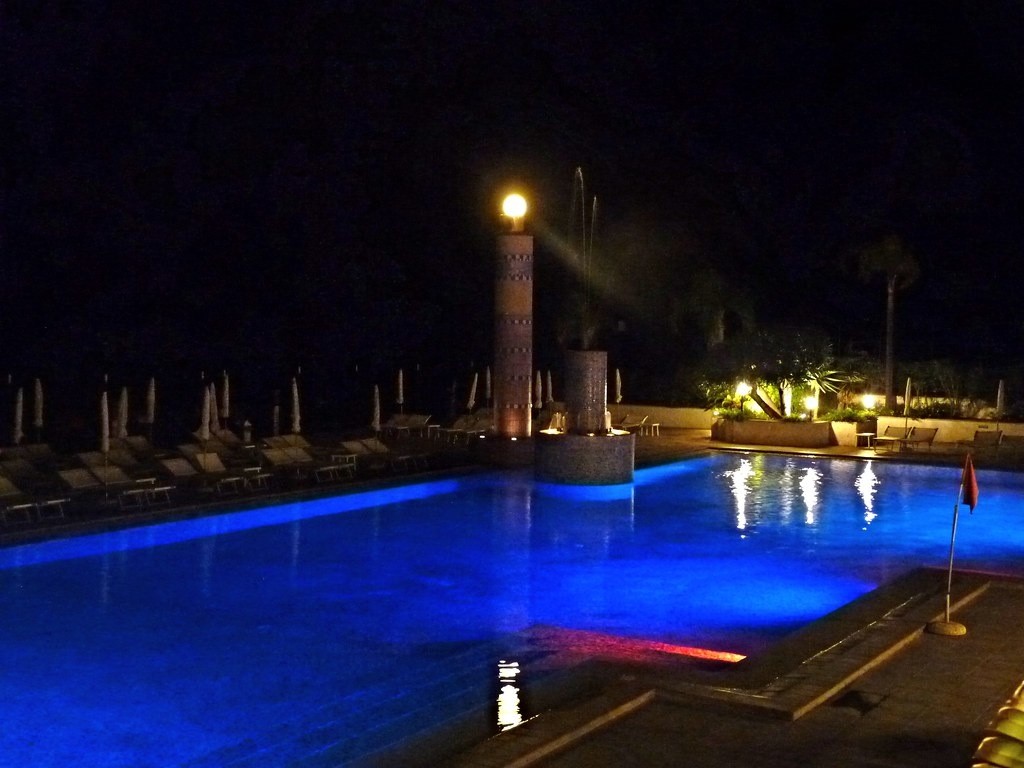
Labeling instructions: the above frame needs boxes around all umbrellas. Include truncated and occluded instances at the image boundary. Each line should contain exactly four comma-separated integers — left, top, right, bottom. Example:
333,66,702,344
616,369,622,422
396,369,404,416
903,378,911,433
10,368,234,452
534,371,554,415
996,380,1004,431
466,367,491,429
372,386,380,442
290,376,300,449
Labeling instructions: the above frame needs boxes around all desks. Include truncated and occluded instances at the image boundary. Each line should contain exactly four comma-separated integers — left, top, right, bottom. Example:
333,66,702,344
639,423,660,437
390,425,410,439
855,433,877,450
421,425,440,438
332,453,357,473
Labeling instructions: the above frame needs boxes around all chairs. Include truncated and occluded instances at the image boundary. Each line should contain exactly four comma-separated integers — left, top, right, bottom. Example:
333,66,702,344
435,416,493,447
956,427,1024,448
872,425,938,453
613,414,649,434
0,429,433,527
380,414,433,441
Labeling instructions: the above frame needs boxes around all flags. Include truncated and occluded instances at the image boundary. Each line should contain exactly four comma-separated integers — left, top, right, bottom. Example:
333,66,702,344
960,454,979,515
